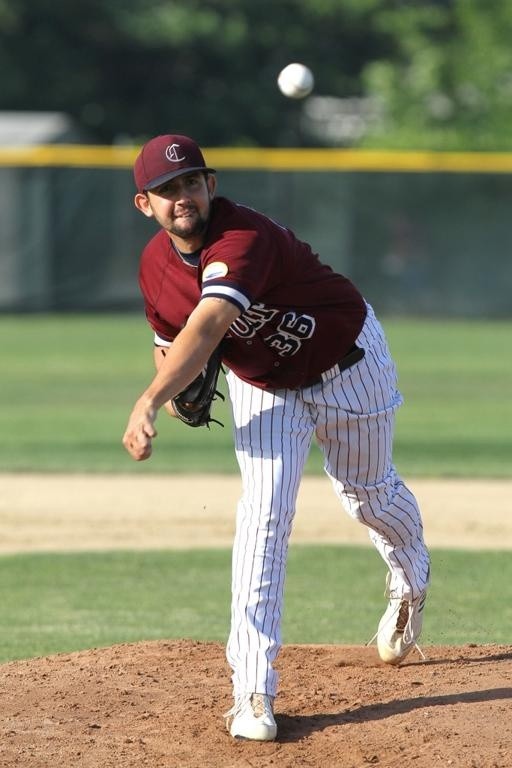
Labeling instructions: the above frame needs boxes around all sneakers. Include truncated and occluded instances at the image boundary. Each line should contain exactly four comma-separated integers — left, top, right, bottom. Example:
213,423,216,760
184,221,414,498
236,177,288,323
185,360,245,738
367,571,429,665
222,693,278,742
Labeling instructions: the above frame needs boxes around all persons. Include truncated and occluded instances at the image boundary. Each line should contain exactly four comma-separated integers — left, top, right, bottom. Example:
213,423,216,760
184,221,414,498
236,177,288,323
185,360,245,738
123,134,432,740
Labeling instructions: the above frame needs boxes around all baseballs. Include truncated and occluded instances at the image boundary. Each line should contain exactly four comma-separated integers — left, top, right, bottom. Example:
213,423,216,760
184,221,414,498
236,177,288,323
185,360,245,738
277,61,315,100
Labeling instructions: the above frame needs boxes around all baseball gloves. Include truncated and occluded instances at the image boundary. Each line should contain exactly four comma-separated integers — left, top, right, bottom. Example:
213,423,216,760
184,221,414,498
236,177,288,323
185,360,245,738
170,352,223,428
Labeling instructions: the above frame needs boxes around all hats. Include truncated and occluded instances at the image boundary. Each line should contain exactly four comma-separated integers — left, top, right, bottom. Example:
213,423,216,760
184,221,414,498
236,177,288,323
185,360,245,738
133,135,216,195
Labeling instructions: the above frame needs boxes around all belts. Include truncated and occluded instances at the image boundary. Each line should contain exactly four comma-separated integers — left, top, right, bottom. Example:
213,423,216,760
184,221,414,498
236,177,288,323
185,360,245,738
288,343,365,390
